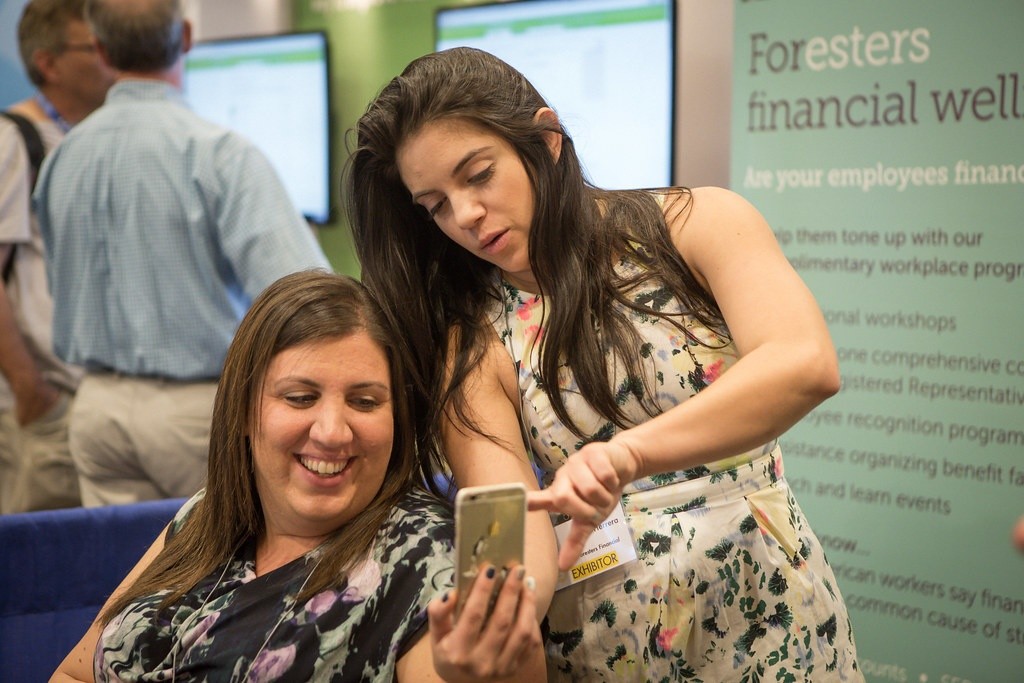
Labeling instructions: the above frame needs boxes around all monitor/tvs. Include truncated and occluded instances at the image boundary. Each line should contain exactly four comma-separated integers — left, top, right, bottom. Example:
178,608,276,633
433,0,678,190
180,27,332,225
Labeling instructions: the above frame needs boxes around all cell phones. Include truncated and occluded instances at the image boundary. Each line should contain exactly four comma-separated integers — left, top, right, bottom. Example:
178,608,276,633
458,483,527,624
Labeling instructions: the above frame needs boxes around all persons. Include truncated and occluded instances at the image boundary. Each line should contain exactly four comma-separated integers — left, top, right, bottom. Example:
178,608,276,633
1,1,339,518
46,268,544,681
340,46,862,681
